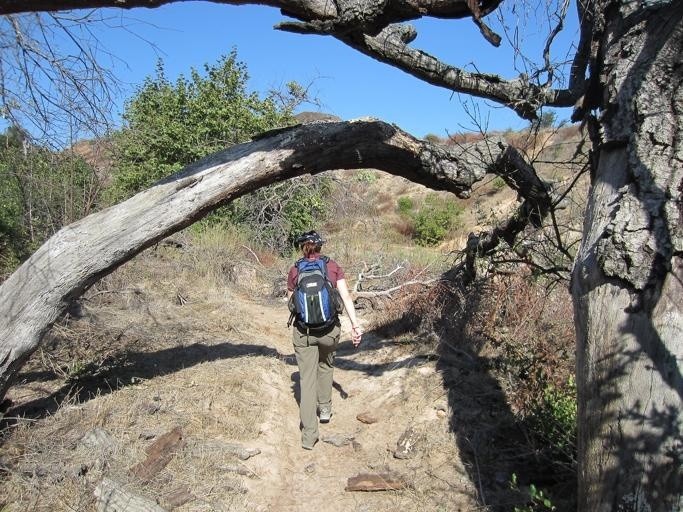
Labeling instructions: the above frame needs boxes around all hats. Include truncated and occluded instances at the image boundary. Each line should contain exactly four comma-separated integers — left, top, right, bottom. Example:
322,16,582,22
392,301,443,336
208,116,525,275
298,230,322,243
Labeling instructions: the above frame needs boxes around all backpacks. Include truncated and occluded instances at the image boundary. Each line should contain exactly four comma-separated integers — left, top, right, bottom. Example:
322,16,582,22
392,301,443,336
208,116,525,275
287,255,341,331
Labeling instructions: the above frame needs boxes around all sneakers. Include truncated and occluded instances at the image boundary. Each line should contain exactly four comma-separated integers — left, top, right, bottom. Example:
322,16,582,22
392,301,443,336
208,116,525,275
320,412,332,421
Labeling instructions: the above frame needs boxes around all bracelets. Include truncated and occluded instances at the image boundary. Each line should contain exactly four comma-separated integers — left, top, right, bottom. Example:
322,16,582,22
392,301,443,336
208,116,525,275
351,326,358,330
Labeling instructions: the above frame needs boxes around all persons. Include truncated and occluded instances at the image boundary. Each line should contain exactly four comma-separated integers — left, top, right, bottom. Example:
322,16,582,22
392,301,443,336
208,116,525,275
285,230,363,452
